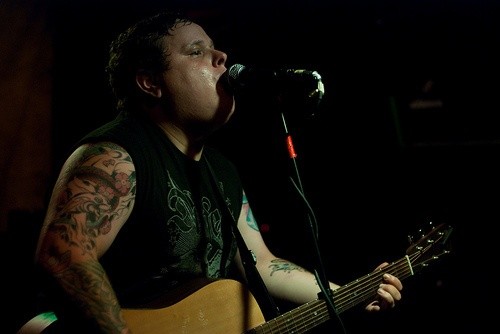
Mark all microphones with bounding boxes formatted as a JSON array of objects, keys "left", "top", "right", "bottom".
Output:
[{"left": 228, "top": 64, "right": 322, "bottom": 85}]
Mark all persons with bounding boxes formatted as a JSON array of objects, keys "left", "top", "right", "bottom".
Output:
[{"left": 34, "top": 15, "right": 403, "bottom": 334}]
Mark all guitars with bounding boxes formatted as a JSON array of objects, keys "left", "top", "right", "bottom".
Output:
[{"left": 17, "top": 219, "right": 469, "bottom": 334}]
